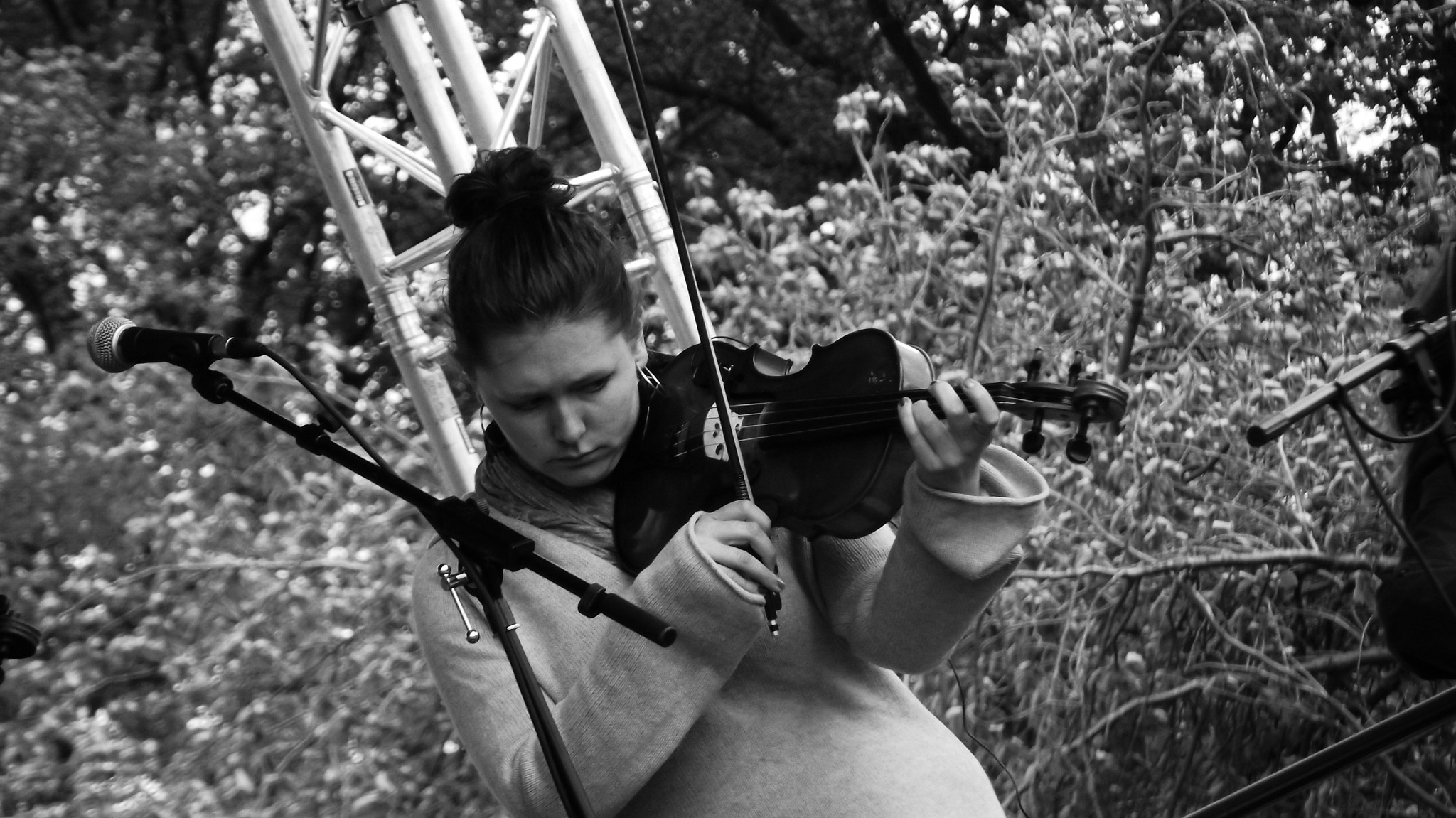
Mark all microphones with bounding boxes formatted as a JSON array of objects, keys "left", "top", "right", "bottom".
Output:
[{"left": 87, "top": 315, "right": 264, "bottom": 373}]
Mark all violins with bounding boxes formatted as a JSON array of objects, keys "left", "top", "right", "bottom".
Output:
[{"left": 610, "top": 326, "right": 1129, "bottom": 577}]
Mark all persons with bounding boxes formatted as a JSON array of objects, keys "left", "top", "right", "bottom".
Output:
[{"left": 408, "top": 146, "right": 1008, "bottom": 818}]
